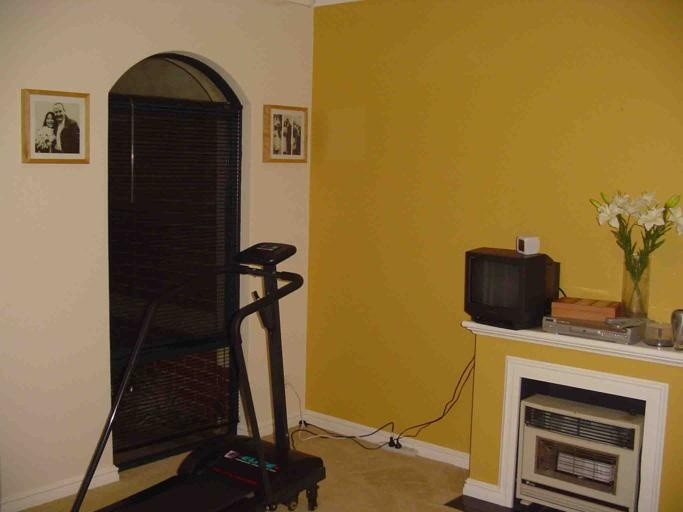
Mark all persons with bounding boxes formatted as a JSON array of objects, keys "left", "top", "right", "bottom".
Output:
[
  {"left": 273, "top": 119, "right": 300, "bottom": 155},
  {"left": 35, "top": 103, "right": 79, "bottom": 153}
]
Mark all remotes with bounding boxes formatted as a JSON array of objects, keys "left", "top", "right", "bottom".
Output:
[{"left": 606, "top": 317, "right": 645, "bottom": 330}]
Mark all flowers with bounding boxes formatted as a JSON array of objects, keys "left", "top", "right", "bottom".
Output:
[{"left": 585, "top": 190, "right": 683, "bottom": 313}]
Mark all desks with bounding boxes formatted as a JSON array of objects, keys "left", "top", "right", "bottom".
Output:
[{"left": 460, "top": 319, "right": 683, "bottom": 511}]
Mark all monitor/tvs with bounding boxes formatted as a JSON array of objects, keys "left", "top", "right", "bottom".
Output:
[{"left": 464, "top": 247, "right": 561, "bottom": 330}]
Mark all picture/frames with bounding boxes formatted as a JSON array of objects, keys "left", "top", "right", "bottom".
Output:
[
  {"left": 20, "top": 88, "right": 91, "bottom": 165},
  {"left": 263, "top": 104, "right": 309, "bottom": 164}
]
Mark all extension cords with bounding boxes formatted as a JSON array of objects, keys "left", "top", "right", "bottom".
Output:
[{"left": 382, "top": 441, "right": 416, "bottom": 457}]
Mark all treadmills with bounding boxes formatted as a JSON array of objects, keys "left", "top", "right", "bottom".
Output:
[{"left": 71, "top": 241, "right": 326, "bottom": 511}]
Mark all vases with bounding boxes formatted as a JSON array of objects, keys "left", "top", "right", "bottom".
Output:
[{"left": 619, "top": 254, "right": 651, "bottom": 318}]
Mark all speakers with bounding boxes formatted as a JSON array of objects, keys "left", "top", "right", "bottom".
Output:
[{"left": 516, "top": 235, "right": 539, "bottom": 256}]
